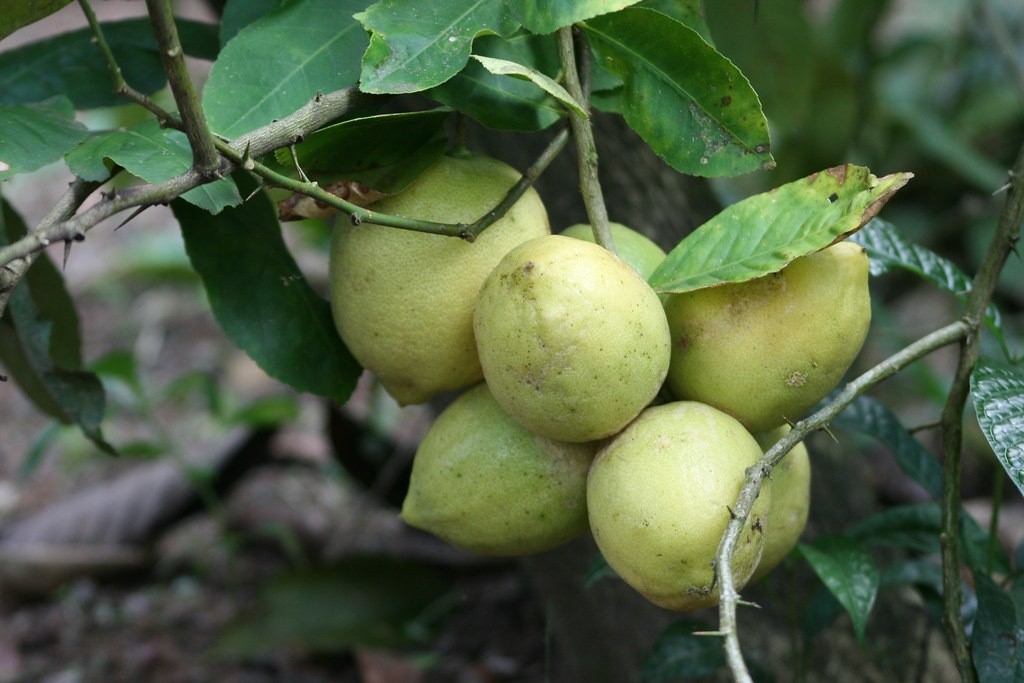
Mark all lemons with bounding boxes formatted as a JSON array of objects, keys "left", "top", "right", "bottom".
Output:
[{"left": 328, "top": 155, "right": 872, "bottom": 615}]
